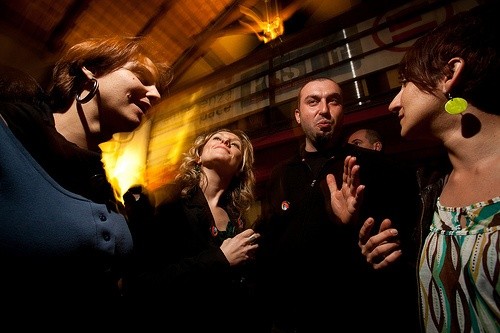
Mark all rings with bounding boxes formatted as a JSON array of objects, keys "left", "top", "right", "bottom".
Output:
[{"left": 246, "top": 236, "right": 257, "bottom": 243}]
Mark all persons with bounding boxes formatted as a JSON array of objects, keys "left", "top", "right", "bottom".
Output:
[
  {"left": 0, "top": 32, "right": 173, "bottom": 333},
  {"left": 123, "top": 20, "right": 500, "bottom": 333}
]
